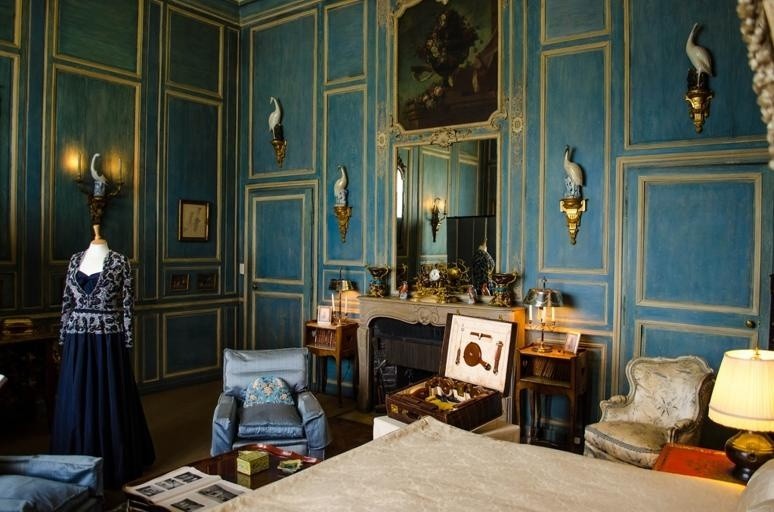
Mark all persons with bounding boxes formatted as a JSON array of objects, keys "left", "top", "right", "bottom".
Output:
[
  {"left": 49, "top": 237, "right": 157, "bottom": 491},
  {"left": 469, "top": 245, "right": 497, "bottom": 293}
]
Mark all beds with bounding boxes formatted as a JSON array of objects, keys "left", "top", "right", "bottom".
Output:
[{"left": 197, "top": 415, "right": 774, "bottom": 512}]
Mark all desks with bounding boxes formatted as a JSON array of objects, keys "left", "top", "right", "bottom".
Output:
[
  {"left": 357, "top": 293, "right": 528, "bottom": 443},
  {"left": 303, "top": 319, "right": 358, "bottom": 409}
]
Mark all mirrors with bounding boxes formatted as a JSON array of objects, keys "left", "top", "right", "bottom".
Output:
[{"left": 390, "top": 133, "right": 501, "bottom": 296}]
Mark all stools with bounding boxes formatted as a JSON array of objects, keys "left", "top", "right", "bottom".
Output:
[{"left": 372, "top": 414, "right": 520, "bottom": 443}]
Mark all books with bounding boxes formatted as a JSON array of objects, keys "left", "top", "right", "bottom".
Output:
[{"left": 124, "top": 464, "right": 252, "bottom": 511}]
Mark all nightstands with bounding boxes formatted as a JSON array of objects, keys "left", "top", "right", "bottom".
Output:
[{"left": 652, "top": 443, "right": 754, "bottom": 486}]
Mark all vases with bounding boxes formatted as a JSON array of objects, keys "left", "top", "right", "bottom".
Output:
[
  {"left": 486, "top": 269, "right": 518, "bottom": 307},
  {"left": 367, "top": 263, "right": 391, "bottom": 297}
]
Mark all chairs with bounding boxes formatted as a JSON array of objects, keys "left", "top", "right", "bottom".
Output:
[
  {"left": 584, "top": 355, "right": 717, "bottom": 469},
  {"left": 209, "top": 348, "right": 333, "bottom": 461},
  {"left": 0, "top": 455, "right": 103, "bottom": 512}
]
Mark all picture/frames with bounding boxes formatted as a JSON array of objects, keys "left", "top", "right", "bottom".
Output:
[
  {"left": 562, "top": 332, "right": 581, "bottom": 354},
  {"left": 317, "top": 305, "right": 333, "bottom": 324},
  {"left": 168, "top": 199, "right": 221, "bottom": 295},
  {"left": 390, "top": 0, "right": 508, "bottom": 134}
]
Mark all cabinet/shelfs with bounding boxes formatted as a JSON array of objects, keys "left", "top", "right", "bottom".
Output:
[
  {"left": 0, "top": 326, "right": 59, "bottom": 431},
  {"left": 516, "top": 342, "right": 592, "bottom": 452}
]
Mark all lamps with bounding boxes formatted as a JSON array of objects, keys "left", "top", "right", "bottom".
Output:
[
  {"left": 328, "top": 268, "right": 354, "bottom": 326},
  {"left": 423, "top": 194, "right": 447, "bottom": 241},
  {"left": 708, "top": 346, "right": 774, "bottom": 481},
  {"left": 239, "top": 255, "right": 245, "bottom": 278},
  {"left": 559, "top": 21, "right": 715, "bottom": 245},
  {"left": 267, "top": 96, "right": 350, "bottom": 241},
  {"left": 75, "top": 151, "right": 124, "bottom": 225},
  {"left": 523, "top": 275, "right": 563, "bottom": 352}
]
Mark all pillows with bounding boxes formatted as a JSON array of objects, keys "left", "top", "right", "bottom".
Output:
[
  {"left": 736, "top": 459, "right": 774, "bottom": 512},
  {"left": 243, "top": 376, "right": 296, "bottom": 406}
]
